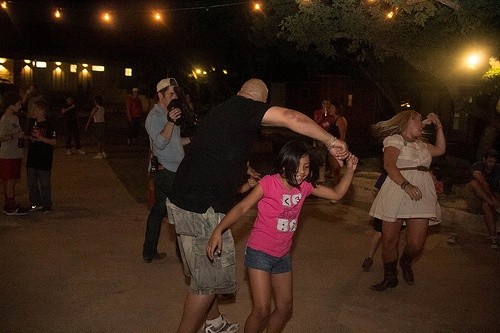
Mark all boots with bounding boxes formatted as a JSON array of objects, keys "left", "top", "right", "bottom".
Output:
[
  {"left": 361, "top": 257, "right": 374, "bottom": 272},
  {"left": 370, "top": 261, "right": 399, "bottom": 291},
  {"left": 399, "top": 252, "right": 415, "bottom": 286}
]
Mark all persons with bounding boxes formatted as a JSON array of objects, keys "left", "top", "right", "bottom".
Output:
[
  {"left": 362, "top": 110, "right": 445, "bottom": 292},
  {"left": 61, "top": 94, "right": 86, "bottom": 154},
  {"left": 125, "top": 87, "right": 143, "bottom": 147},
  {"left": 143, "top": 78, "right": 195, "bottom": 262},
  {"left": 85, "top": 95, "right": 108, "bottom": 159},
  {"left": 206, "top": 139, "right": 359, "bottom": 333},
  {"left": 311, "top": 97, "right": 347, "bottom": 204},
  {"left": 164, "top": 78, "right": 349, "bottom": 333},
  {"left": 236, "top": 150, "right": 275, "bottom": 192},
  {"left": 462, "top": 149, "right": 500, "bottom": 249},
  {"left": 0, "top": 84, "right": 57, "bottom": 216}
]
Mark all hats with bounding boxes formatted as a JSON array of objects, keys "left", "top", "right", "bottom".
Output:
[
  {"left": 156, "top": 77, "right": 179, "bottom": 93},
  {"left": 132, "top": 88, "right": 138, "bottom": 91}
]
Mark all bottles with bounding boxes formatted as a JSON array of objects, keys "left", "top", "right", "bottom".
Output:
[
  {"left": 325, "top": 107, "right": 327, "bottom": 116},
  {"left": 34, "top": 121, "right": 39, "bottom": 139}
]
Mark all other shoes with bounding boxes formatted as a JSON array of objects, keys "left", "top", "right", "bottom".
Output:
[
  {"left": 76, "top": 149, "right": 86, "bottom": 155},
  {"left": 103, "top": 152, "right": 109, "bottom": 158},
  {"left": 202, "top": 318, "right": 239, "bottom": 333},
  {"left": 43, "top": 207, "right": 52, "bottom": 214},
  {"left": 27, "top": 203, "right": 42, "bottom": 212},
  {"left": 66, "top": 148, "right": 72, "bottom": 155},
  {"left": 145, "top": 251, "right": 167, "bottom": 263},
  {"left": 447, "top": 232, "right": 459, "bottom": 244},
  {"left": 2, "top": 207, "right": 28, "bottom": 216},
  {"left": 488, "top": 236, "right": 498, "bottom": 248},
  {"left": 93, "top": 155, "right": 103, "bottom": 159}
]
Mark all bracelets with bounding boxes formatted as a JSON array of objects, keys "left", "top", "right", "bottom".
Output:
[
  {"left": 402, "top": 181, "right": 409, "bottom": 189},
  {"left": 326, "top": 137, "right": 337, "bottom": 151}
]
